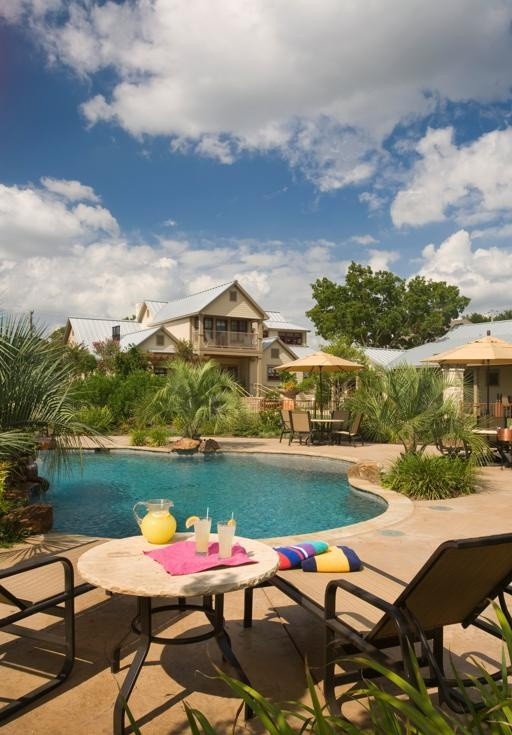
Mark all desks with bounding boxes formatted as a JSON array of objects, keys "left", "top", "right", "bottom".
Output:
[{"left": 78, "top": 531, "right": 280, "bottom": 735}]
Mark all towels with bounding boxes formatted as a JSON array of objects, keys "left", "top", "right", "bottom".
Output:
[
  {"left": 301, "top": 546, "right": 361, "bottom": 573},
  {"left": 273, "top": 539, "right": 329, "bottom": 571}
]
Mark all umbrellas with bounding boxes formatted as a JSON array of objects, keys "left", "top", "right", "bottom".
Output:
[
  {"left": 272, "top": 350, "right": 366, "bottom": 441},
  {"left": 419, "top": 328, "right": 512, "bottom": 456}
]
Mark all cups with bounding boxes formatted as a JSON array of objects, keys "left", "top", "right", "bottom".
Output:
[
  {"left": 193, "top": 517, "right": 212, "bottom": 557},
  {"left": 216, "top": 520, "right": 236, "bottom": 560}
]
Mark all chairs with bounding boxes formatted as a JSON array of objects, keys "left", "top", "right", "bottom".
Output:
[
  {"left": 244, "top": 532, "right": 512, "bottom": 735},
  {"left": 278, "top": 410, "right": 364, "bottom": 449},
  {"left": 432, "top": 416, "right": 512, "bottom": 468},
  {"left": 1, "top": 538, "right": 114, "bottom": 722}
]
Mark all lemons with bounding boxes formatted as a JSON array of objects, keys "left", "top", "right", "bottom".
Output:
[
  {"left": 227, "top": 519, "right": 236, "bottom": 526},
  {"left": 185, "top": 516, "right": 199, "bottom": 528}
]
